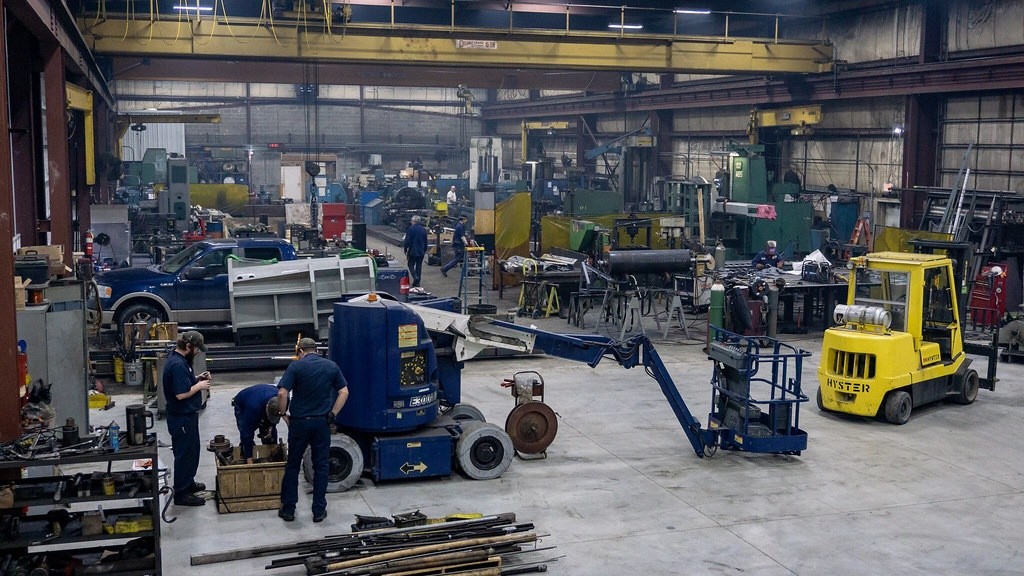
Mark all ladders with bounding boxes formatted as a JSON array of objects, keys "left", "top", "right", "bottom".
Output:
[
  {"left": 458, "top": 246, "right": 489, "bottom": 316},
  {"left": 844, "top": 216, "right": 872, "bottom": 259}
]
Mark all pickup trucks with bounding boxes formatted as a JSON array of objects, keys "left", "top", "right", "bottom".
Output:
[{"left": 85, "top": 238, "right": 411, "bottom": 344}]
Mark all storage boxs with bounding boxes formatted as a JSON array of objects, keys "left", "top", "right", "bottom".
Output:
[
  {"left": 14, "top": 253, "right": 51, "bottom": 280},
  {"left": 15, "top": 260, "right": 49, "bottom": 283},
  {"left": 14, "top": 276, "right": 31, "bottom": 311},
  {"left": 217, "top": 442, "right": 287, "bottom": 512},
  {"left": 124, "top": 321, "right": 146, "bottom": 349},
  {"left": 21, "top": 245, "right": 64, "bottom": 263},
  {"left": 157, "top": 321, "right": 178, "bottom": 345}
]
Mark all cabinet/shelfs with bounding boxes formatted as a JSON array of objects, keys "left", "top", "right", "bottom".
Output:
[{"left": 0, "top": 432, "right": 161, "bottom": 575}]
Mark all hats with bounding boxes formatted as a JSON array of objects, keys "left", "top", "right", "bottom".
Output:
[
  {"left": 266, "top": 397, "right": 281, "bottom": 423},
  {"left": 296, "top": 338, "right": 316, "bottom": 356},
  {"left": 451, "top": 186, "right": 455, "bottom": 188},
  {"left": 767, "top": 240, "right": 777, "bottom": 248},
  {"left": 183, "top": 331, "right": 208, "bottom": 352}
]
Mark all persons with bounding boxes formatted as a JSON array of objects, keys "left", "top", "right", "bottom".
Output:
[
  {"left": 441, "top": 215, "right": 473, "bottom": 277},
  {"left": 278, "top": 338, "right": 348, "bottom": 522},
  {"left": 404, "top": 216, "right": 428, "bottom": 286},
  {"left": 234, "top": 384, "right": 291, "bottom": 464},
  {"left": 447, "top": 186, "right": 459, "bottom": 205},
  {"left": 751, "top": 241, "right": 784, "bottom": 269},
  {"left": 163, "top": 331, "right": 212, "bottom": 506}
]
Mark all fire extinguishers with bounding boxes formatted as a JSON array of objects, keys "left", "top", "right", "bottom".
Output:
[
  {"left": 400, "top": 271, "right": 409, "bottom": 294},
  {"left": 85, "top": 229, "right": 95, "bottom": 255}
]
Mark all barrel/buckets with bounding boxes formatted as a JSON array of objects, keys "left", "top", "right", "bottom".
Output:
[{"left": 113, "top": 354, "right": 144, "bottom": 386}]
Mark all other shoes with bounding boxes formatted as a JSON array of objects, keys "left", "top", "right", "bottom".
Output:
[
  {"left": 440, "top": 268, "right": 448, "bottom": 277},
  {"left": 313, "top": 510, "right": 327, "bottom": 522},
  {"left": 193, "top": 482, "right": 206, "bottom": 494},
  {"left": 175, "top": 494, "right": 206, "bottom": 506},
  {"left": 412, "top": 278, "right": 419, "bottom": 287},
  {"left": 278, "top": 513, "right": 294, "bottom": 521}
]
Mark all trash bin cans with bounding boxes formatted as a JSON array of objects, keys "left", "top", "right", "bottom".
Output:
[{"left": 352, "top": 223, "right": 366, "bottom": 252}]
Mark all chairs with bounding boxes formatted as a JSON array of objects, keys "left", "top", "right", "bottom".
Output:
[{"left": 887, "top": 307, "right": 904, "bottom": 333}]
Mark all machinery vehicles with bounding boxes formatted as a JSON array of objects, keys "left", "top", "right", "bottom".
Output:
[
  {"left": 816, "top": 249, "right": 1003, "bottom": 424},
  {"left": 301, "top": 292, "right": 814, "bottom": 494}
]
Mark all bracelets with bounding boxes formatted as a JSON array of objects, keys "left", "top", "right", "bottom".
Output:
[{"left": 279, "top": 412, "right": 286, "bottom": 416}]
